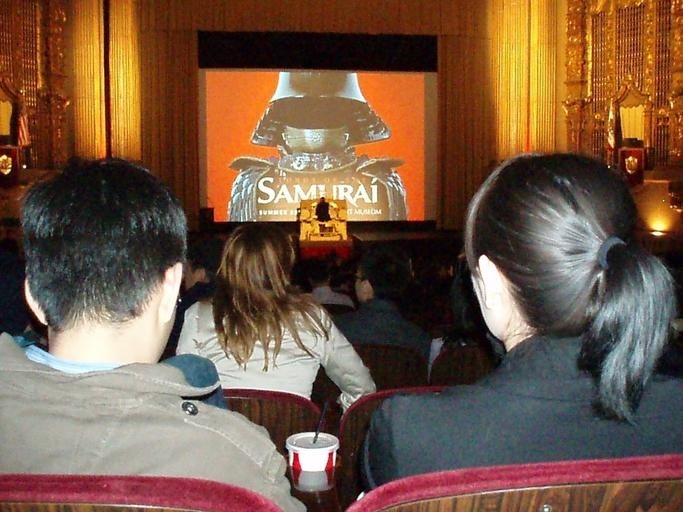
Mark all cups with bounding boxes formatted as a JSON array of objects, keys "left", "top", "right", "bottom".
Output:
[
  {"left": 282, "top": 430, "right": 341, "bottom": 470},
  {"left": 290, "top": 470, "right": 339, "bottom": 495}
]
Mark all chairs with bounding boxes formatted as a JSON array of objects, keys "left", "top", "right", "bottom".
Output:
[
  {"left": 221, "top": 388, "right": 325, "bottom": 457},
  {"left": 344, "top": 454, "right": 682, "bottom": 512},
  {"left": 339, "top": 345, "right": 494, "bottom": 457},
  {"left": 0, "top": 473, "right": 282, "bottom": 512}
]
